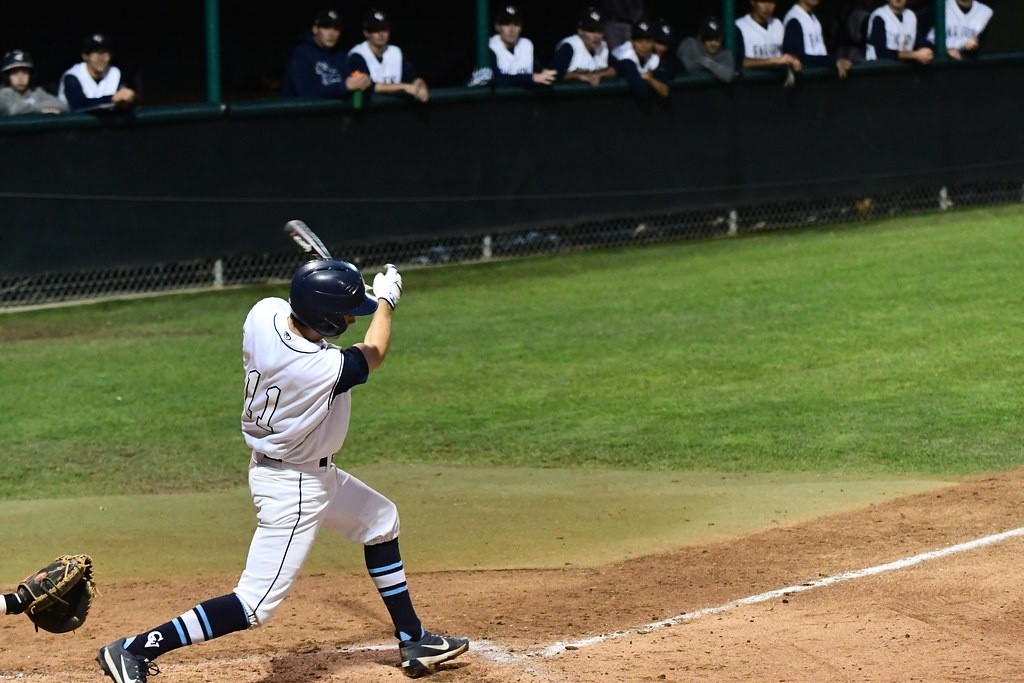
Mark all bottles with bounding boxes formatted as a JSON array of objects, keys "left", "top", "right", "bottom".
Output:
[{"left": 353, "top": 69, "right": 363, "bottom": 108}]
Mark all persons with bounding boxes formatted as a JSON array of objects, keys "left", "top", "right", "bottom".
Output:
[
  {"left": 0, "top": 49, "right": 68, "bottom": 116},
  {"left": 924, "top": 0, "right": 994, "bottom": 60},
  {"left": 466, "top": 5, "right": 557, "bottom": 86},
  {"left": 865, "top": 0, "right": 933, "bottom": 64},
  {"left": 58, "top": 33, "right": 135, "bottom": 112},
  {"left": 605, "top": 12, "right": 736, "bottom": 103},
  {"left": 734, "top": 0, "right": 802, "bottom": 86},
  {"left": 97, "top": 257, "right": 469, "bottom": 683},
  {"left": 782, "top": 0, "right": 852, "bottom": 77},
  {"left": 553, "top": 9, "right": 621, "bottom": 88},
  {"left": 0, "top": 554, "right": 96, "bottom": 633},
  {"left": 347, "top": 12, "right": 429, "bottom": 103},
  {"left": 284, "top": 10, "right": 375, "bottom": 100}
]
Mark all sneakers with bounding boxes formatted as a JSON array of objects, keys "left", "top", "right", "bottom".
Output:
[
  {"left": 94, "top": 638, "right": 159, "bottom": 683},
  {"left": 398, "top": 631, "right": 469, "bottom": 679}
]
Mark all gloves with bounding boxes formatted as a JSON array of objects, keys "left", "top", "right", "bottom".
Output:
[{"left": 373, "top": 263, "right": 403, "bottom": 310}]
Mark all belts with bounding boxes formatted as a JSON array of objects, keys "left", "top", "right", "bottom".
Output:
[{"left": 263, "top": 453, "right": 334, "bottom": 467}]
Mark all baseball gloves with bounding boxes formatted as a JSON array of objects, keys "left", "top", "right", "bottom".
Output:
[{"left": 17, "top": 554, "right": 94, "bottom": 634}]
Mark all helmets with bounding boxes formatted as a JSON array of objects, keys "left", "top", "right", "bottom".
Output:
[{"left": 288, "top": 260, "right": 379, "bottom": 338}]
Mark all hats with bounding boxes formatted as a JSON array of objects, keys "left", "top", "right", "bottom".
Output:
[
  {"left": 495, "top": 6, "right": 522, "bottom": 23},
  {"left": 82, "top": 32, "right": 112, "bottom": 53},
  {"left": 699, "top": 17, "right": 723, "bottom": 37},
  {"left": 653, "top": 19, "right": 674, "bottom": 42},
  {"left": 630, "top": 21, "right": 653, "bottom": 40},
  {"left": 0, "top": 49, "right": 33, "bottom": 72},
  {"left": 313, "top": 10, "right": 345, "bottom": 31},
  {"left": 574, "top": 6, "right": 606, "bottom": 30},
  {"left": 361, "top": 10, "right": 391, "bottom": 31}
]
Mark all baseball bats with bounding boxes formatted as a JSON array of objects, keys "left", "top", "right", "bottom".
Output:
[{"left": 284, "top": 220, "right": 332, "bottom": 261}]
